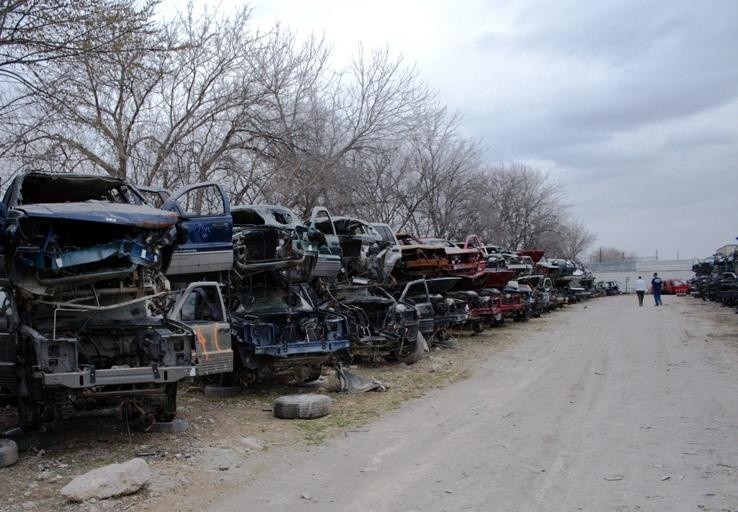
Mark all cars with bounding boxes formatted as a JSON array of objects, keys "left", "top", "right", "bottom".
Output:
[
  {"left": 0, "top": 168, "right": 620, "bottom": 430},
  {"left": 647, "top": 249, "right": 738, "bottom": 314}
]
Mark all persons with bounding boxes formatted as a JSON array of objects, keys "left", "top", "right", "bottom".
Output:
[
  {"left": 650, "top": 272, "right": 663, "bottom": 307},
  {"left": 634, "top": 275, "right": 648, "bottom": 307}
]
